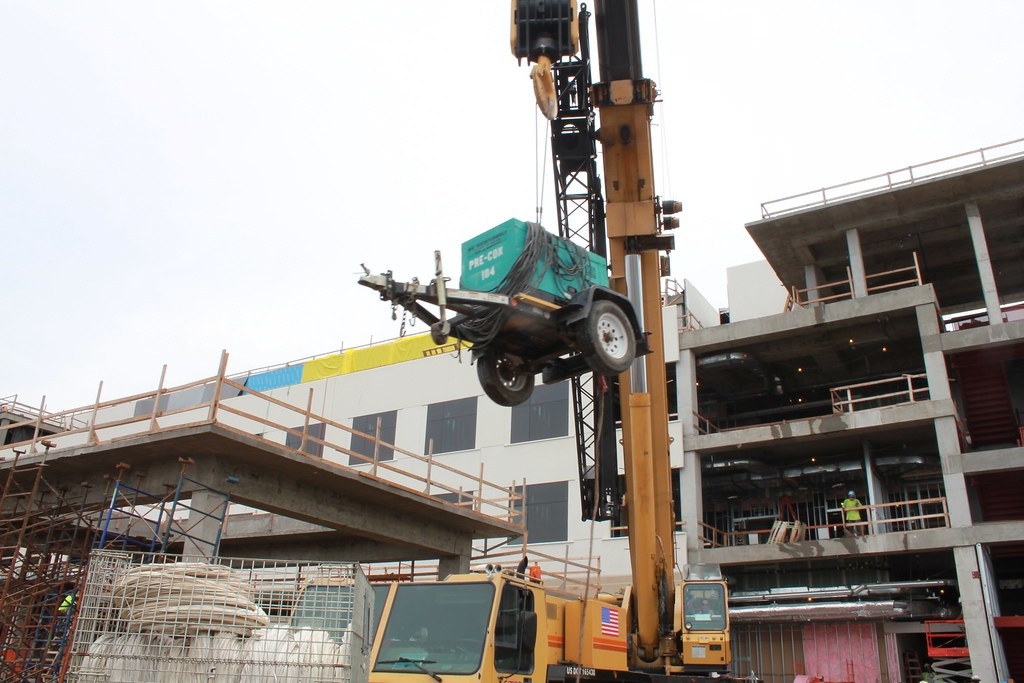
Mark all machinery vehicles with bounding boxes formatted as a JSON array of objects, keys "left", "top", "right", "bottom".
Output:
[{"left": 349, "top": 0, "right": 734, "bottom": 683}]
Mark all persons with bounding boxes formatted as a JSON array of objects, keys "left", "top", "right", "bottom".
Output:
[
  {"left": 842, "top": 490, "right": 865, "bottom": 537},
  {"left": 53, "top": 592, "right": 74, "bottom": 640}
]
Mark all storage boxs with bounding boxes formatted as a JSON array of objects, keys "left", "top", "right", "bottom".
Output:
[{"left": 460, "top": 218, "right": 610, "bottom": 306}]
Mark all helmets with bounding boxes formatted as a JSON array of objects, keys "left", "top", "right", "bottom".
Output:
[{"left": 848, "top": 490, "right": 856, "bottom": 497}]
